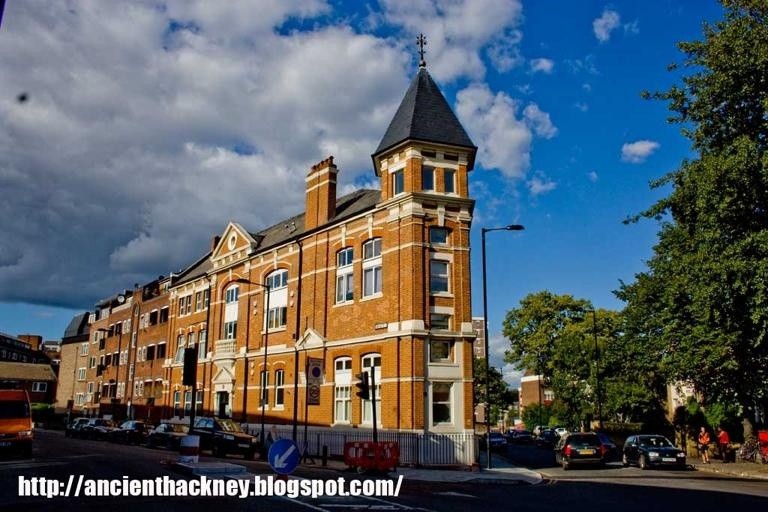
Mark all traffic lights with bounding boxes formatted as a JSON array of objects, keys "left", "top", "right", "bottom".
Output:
[{"left": 354, "top": 371, "right": 369, "bottom": 401}]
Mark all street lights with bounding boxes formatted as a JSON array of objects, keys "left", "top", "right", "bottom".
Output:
[
  {"left": 569, "top": 307, "right": 604, "bottom": 429},
  {"left": 235, "top": 278, "right": 270, "bottom": 461},
  {"left": 481, "top": 225, "right": 525, "bottom": 469},
  {"left": 97, "top": 328, "right": 122, "bottom": 423}
]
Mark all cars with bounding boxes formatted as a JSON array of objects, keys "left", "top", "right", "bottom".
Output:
[
  {"left": 64, "top": 417, "right": 255, "bottom": 461},
  {"left": 480, "top": 425, "right": 685, "bottom": 470}
]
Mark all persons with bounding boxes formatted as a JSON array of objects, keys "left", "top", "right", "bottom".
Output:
[
  {"left": 717, "top": 425, "right": 731, "bottom": 461},
  {"left": 697, "top": 426, "right": 710, "bottom": 464}
]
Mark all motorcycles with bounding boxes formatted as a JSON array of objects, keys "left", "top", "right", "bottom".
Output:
[{"left": 739, "top": 436, "right": 768, "bottom": 464}]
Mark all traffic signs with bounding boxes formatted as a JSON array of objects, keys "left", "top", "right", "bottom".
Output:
[{"left": 307, "top": 383, "right": 320, "bottom": 405}]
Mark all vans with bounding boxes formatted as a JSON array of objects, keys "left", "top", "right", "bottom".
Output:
[{"left": 0, "top": 390, "right": 34, "bottom": 453}]
